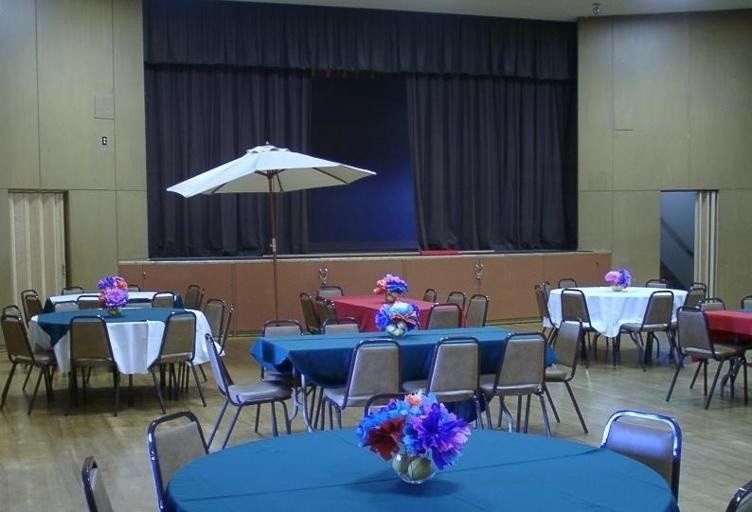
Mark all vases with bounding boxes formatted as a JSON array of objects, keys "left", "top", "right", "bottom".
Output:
[{"left": 392, "top": 453, "right": 436, "bottom": 485}]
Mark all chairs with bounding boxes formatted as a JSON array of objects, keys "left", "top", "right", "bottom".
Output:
[
  {"left": 725, "top": 480, "right": 752, "bottom": 512},
  {"left": 82, "top": 456, "right": 116, "bottom": 512},
  {"left": 600, "top": 410, "right": 682, "bottom": 503},
  {"left": 147, "top": 410, "right": 210, "bottom": 511}
]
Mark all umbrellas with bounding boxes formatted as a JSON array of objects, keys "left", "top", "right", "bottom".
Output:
[{"left": 167, "top": 141, "right": 376, "bottom": 322}]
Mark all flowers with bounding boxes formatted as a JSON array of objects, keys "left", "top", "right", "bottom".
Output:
[
  {"left": 604, "top": 268, "right": 631, "bottom": 288},
  {"left": 355, "top": 388, "right": 473, "bottom": 481}
]
